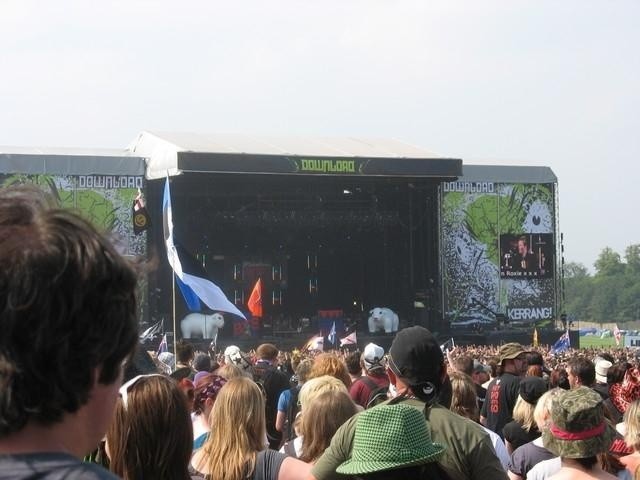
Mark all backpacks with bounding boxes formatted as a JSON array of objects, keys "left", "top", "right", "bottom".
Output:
[
  {"left": 246, "top": 365, "right": 276, "bottom": 415},
  {"left": 359, "top": 376, "right": 389, "bottom": 410}
]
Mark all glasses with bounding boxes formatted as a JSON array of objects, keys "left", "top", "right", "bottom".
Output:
[{"left": 118, "top": 374, "right": 161, "bottom": 411}]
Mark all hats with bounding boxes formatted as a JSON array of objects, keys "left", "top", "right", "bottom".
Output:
[
  {"left": 591, "top": 356, "right": 613, "bottom": 384},
  {"left": 473, "top": 359, "right": 492, "bottom": 372},
  {"left": 336, "top": 404, "right": 447, "bottom": 475},
  {"left": 194, "top": 355, "right": 210, "bottom": 371},
  {"left": 158, "top": 352, "right": 176, "bottom": 367},
  {"left": 383, "top": 326, "right": 445, "bottom": 402},
  {"left": 363, "top": 342, "right": 386, "bottom": 371},
  {"left": 609, "top": 368, "right": 640, "bottom": 413},
  {"left": 495, "top": 342, "right": 532, "bottom": 366},
  {"left": 519, "top": 376, "right": 548, "bottom": 404},
  {"left": 542, "top": 386, "right": 618, "bottom": 459},
  {"left": 192, "top": 371, "right": 209, "bottom": 385}
]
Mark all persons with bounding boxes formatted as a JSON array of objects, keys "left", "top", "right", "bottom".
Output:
[
  {"left": 508, "top": 235, "right": 540, "bottom": 273},
  {"left": 82, "top": 326, "right": 640, "bottom": 480},
  {"left": 0, "top": 186, "right": 139, "bottom": 479}
]
